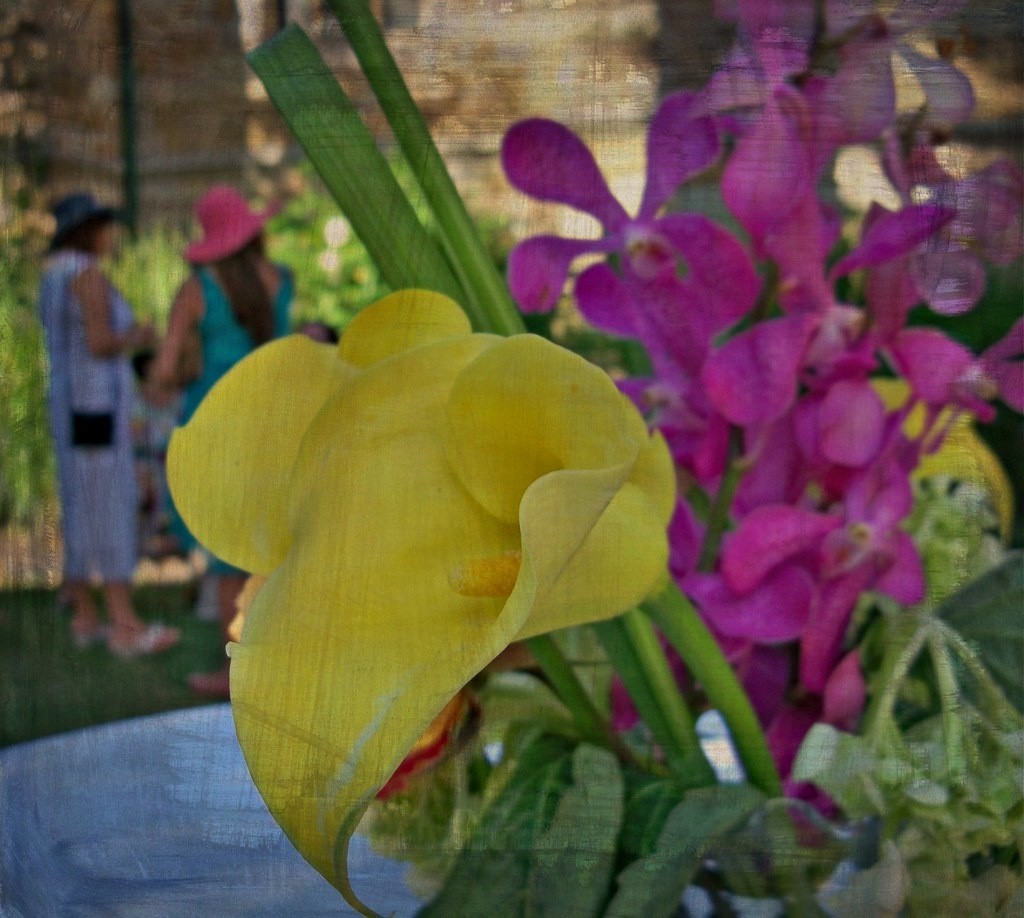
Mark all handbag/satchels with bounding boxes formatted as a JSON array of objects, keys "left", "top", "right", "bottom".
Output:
[{"left": 71, "top": 410, "right": 116, "bottom": 450}]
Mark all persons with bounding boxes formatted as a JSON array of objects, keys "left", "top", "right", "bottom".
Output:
[
  {"left": 129, "top": 331, "right": 183, "bottom": 459},
  {"left": 295, "top": 319, "right": 340, "bottom": 345},
  {"left": 32, "top": 189, "right": 180, "bottom": 656},
  {"left": 154, "top": 185, "right": 302, "bottom": 632}
]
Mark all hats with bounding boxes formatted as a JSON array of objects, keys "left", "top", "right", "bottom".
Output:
[
  {"left": 48, "top": 191, "right": 119, "bottom": 251},
  {"left": 185, "top": 187, "right": 281, "bottom": 262}
]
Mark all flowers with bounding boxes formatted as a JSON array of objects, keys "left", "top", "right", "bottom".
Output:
[{"left": 168, "top": 0, "right": 1024, "bottom": 918}]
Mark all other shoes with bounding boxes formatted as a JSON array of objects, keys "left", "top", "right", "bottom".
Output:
[
  {"left": 110, "top": 626, "right": 178, "bottom": 661},
  {"left": 189, "top": 661, "right": 229, "bottom": 701},
  {"left": 74, "top": 623, "right": 107, "bottom": 651}
]
